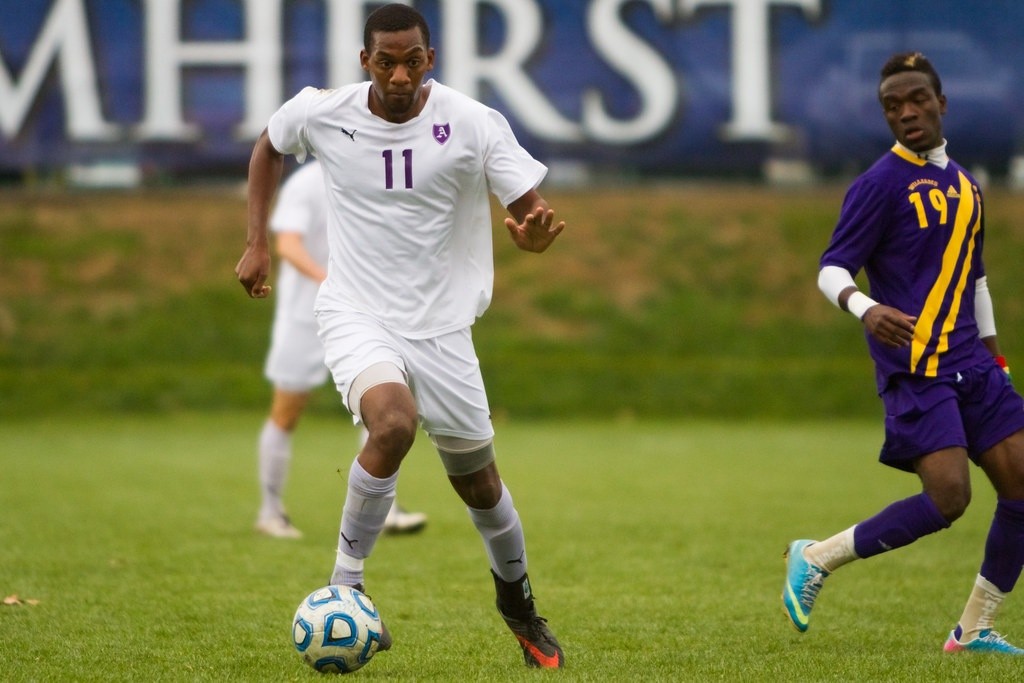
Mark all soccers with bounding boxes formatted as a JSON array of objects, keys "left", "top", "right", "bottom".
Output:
[{"left": 292, "top": 585, "right": 383, "bottom": 674}]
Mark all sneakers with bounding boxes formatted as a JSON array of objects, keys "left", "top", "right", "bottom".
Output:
[
  {"left": 329, "top": 578, "right": 393, "bottom": 654},
  {"left": 380, "top": 507, "right": 427, "bottom": 534},
  {"left": 495, "top": 602, "right": 568, "bottom": 675},
  {"left": 257, "top": 517, "right": 306, "bottom": 541},
  {"left": 781, "top": 536, "right": 831, "bottom": 633},
  {"left": 942, "top": 626, "right": 1023, "bottom": 660}
]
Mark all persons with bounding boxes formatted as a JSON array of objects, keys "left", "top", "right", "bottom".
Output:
[
  {"left": 235, "top": 3, "right": 567, "bottom": 674},
  {"left": 776, "top": 54, "right": 1024, "bottom": 656},
  {"left": 253, "top": 157, "right": 429, "bottom": 539}
]
